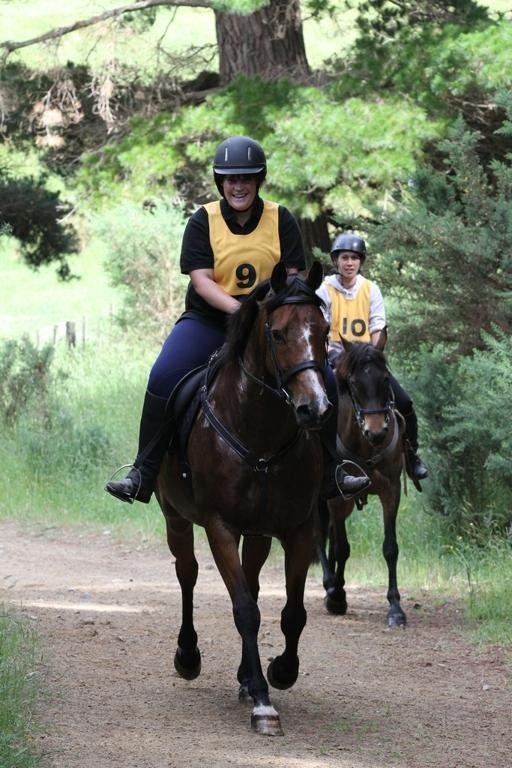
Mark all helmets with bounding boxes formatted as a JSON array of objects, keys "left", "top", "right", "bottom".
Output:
[
  {"left": 212, "top": 135, "right": 266, "bottom": 198},
  {"left": 329, "top": 232, "right": 368, "bottom": 261}
]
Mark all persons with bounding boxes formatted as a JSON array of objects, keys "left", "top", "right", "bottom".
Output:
[
  {"left": 314, "top": 232, "right": 429, "bottom": 480},
  {"left": 105, "top": 134, "right": 370, "bottom": 505}
]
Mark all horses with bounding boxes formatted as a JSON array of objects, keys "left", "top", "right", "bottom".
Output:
[
  {"left": 307, "top": 324, "right": 408, "bottom": 632},
  {"left": 150, "top": 257, "right": 333, "bottom": 738}
]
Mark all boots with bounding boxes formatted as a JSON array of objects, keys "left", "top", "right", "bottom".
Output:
[
  {"left": 312, "top": 399, "right": 374, "bottom": 496},
  {"left": 102, "top": 387, "right": 178, "bottom": 505},
  {"left": 397, "top": 409, "right": 430, "bottom": 482}
]
